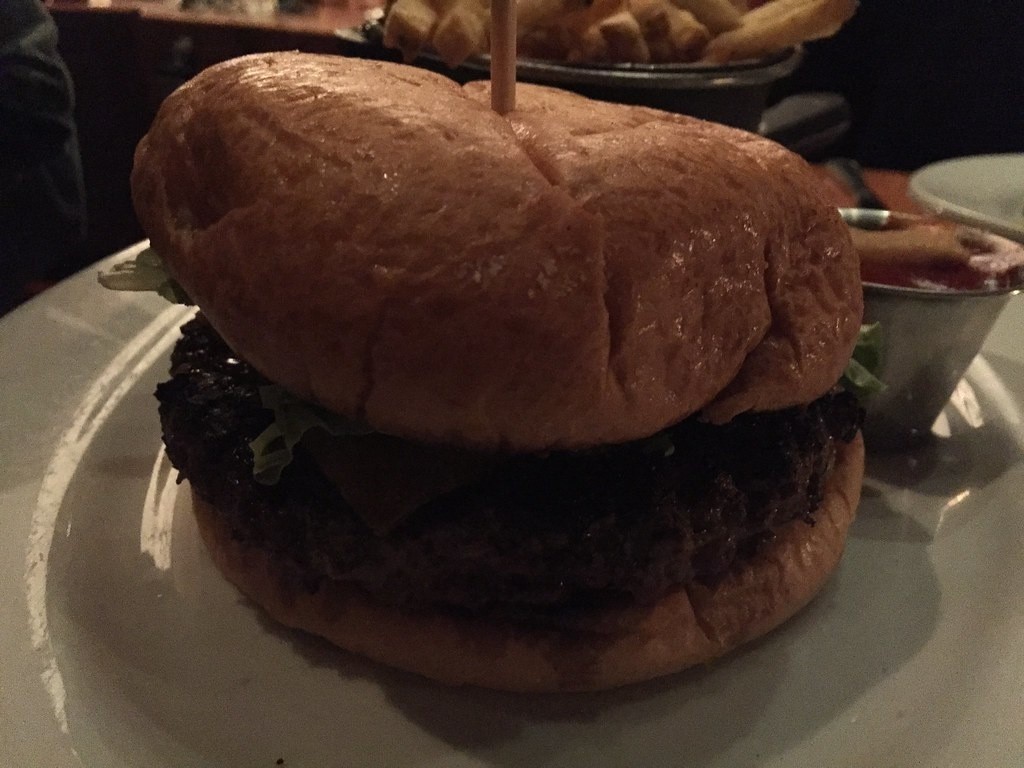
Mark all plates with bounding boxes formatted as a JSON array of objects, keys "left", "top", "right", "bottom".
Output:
[
  {"left": 0, "top": 237, "right": 1024, "bottom": 768},
  {"left": 907, "top": 152, "right": 1024, "bottom": 241}
]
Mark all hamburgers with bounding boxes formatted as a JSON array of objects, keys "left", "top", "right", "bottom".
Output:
[{"left": 96, "top": 53, "right": 887, "bottom": 694}]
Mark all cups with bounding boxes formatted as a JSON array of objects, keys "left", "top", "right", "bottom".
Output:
[{"left": 836, "top": 209, "right": 1024, "bottom": 431}]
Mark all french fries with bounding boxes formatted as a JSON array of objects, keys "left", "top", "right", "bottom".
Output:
[{"left": 380, "top": 0, "right": 855, "bottom": 64}]
